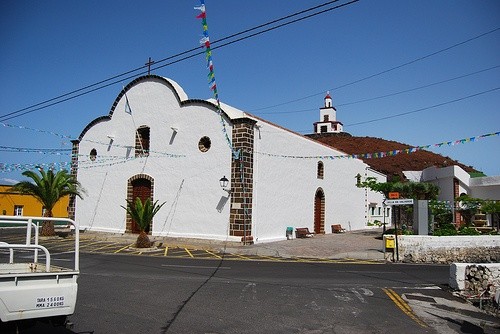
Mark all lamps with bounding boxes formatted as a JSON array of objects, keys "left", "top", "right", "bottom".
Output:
[{"left": 219, "top": 176, "right": 230, "bottom": 188}]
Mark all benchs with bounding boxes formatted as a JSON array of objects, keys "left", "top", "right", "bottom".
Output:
[
  {"left": 295, "top": 228, "right": 316, "bottom": 239},
  {"left": 331, "top": 224, "right": 346, "bottom": 234}
]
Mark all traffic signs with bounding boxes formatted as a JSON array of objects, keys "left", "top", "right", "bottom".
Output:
[{"left": 383, "top": 199, "right": 415, "bottom": 205}]
